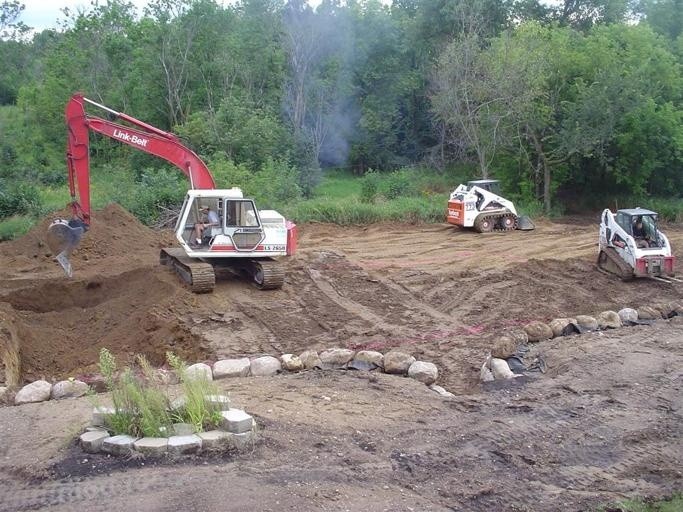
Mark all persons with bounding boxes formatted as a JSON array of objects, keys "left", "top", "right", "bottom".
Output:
[
  {"left": 606, "top": 219, "right": 627, "bottom": 249},
  {"left": 191, "top": 205, "right": 221, "bottom": 250},
  {"left": 633, "top": 218, "right": 655, "bottom": 249}
]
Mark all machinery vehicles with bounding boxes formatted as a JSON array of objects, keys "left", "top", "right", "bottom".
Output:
[
  {"left": 444, "top": 177, "right": 533, "bottom": 235},
  {"left": 592, "top": 205, "right": 682, "bottom": 285},
  {"left": 45, "top": 89, "right": 301, "bottom": 297}
]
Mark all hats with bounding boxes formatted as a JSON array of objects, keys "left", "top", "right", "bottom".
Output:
[{"left": 198, "top": 205, "right": 208, "bottom": 211}]
